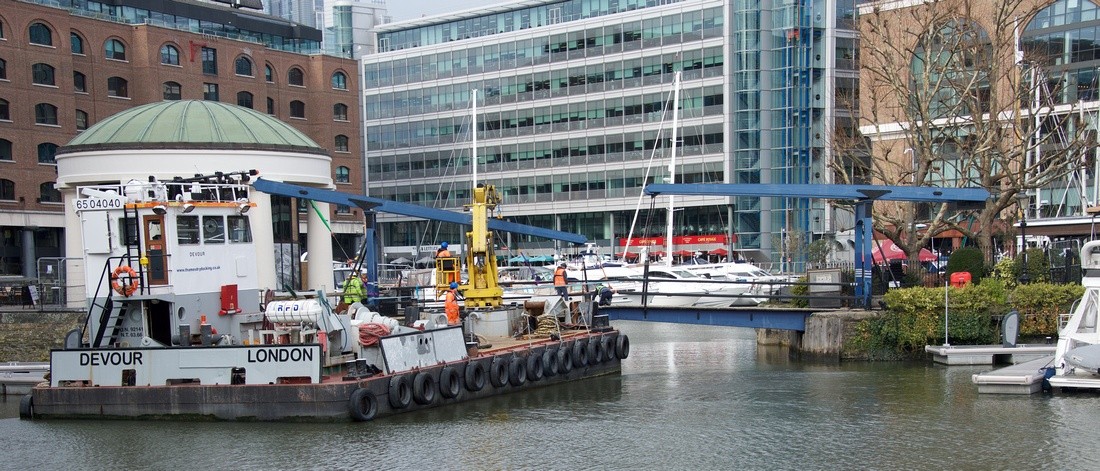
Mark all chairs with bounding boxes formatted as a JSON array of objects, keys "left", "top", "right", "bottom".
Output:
[{"left": 0, "top": 286, "right": 22, "bottom": 306}]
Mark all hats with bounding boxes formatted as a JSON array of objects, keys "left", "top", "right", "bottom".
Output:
[{"left": 561, "top": 263, "right": 567, "bottom": 268}]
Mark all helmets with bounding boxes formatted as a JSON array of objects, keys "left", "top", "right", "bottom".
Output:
[
  {"left": 450, "top": 283, "right": 458, "bottom": 288},
  {"left": 442, "top": 242, "right": 447, "bottom": 248},
  {"left": 349, "top": 271, "right": 356, "bottom": 275},
  {"left": 362, "top": 269, "right": 367, "bottom": 273}
]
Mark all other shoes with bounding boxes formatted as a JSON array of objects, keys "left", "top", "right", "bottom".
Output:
[
  {"left": 605, "top": 298, "right": 609, "bottom": 305},
  {"left": 609, "top": 298, "right": 612, "bottom": 304}
]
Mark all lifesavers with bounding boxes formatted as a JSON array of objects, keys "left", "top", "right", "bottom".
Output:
[
  {"left": 348, "top": 388, "right": 378, "bottom": 421},
  {"left": 616, "top": 334, "right": 629, "bottom": 359},
  {"left": 413, "top": 372, "right": 436, "bottom": 405},
  {"left": 112, "top": 267, "right": 137, "bottom": 296},
  {"left": 556, "top": 348, "right": 573, "bottom": 374},
  {"left": 19, "top": 393, "right": 33, "bottom": 420},
  {"left": 586, "top": 341, "right": 604, "bottom": 365},
  {"left": 389, "top": 376, "right": 412, "bottom": 410},
  {"left": 526, "top": 355, "right": 545, "bottom": 381},
  {"left": 573, "top": 341, "right": 589, "bottom": 369},
  {"left": 600, "top": 336, "right": 615, "bottom": 361},
  {"left": 490, "top": 357, "right": 510, "bottom": 388},
  {"left": 543, "top": 349, "right": 560, "bottom": 378},
  {"left": 465, "top": 360, "right": 485, "bottom": 391},
  {"left": 509, "top": 359, "right": 527, "bottom": 386},
  {"left": 439, "top": 367, "right": 461, "bottom": 399}
]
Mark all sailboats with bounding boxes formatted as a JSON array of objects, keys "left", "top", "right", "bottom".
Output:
[
  {"left": 402, "top": 68, "right": 792, "bottom": 307},
  {"left": 1012, "top": 60, "right": 1100, "bottom": 236}
]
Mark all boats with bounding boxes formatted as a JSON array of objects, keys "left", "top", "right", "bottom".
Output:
[
  {"left": 1038, "top": 239, "right": 1100, "bottom": 395},
  {"left": 18, "top": 181, "right": 629, "bottom": 425}
]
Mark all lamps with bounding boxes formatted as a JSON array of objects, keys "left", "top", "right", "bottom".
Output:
[{"left": 153, "top": 202, "right": 252, "bottom": 215}]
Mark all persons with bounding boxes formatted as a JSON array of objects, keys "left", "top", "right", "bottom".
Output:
[
  {"left": 445, "top": 283, "right": 459, "bottom": 325},
  {"left": 591, "top": 286, "right": 612, "bottom": 306},
  {"left": 343, "top": 273, "right": 369, "bottom": 307},
  {"left": 553, "top": 263, "right": 570, "bottom": 302},
  {"left": 436, "top": 242, "right": 450, "bottom": 258}
]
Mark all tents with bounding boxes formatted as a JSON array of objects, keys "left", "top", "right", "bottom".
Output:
[
  {"left": 862, "top": 237, "right": 937, "bottom": 267},
  {"left": 509, "top": 255, "right": 554, "bottom": 268},
  {"left": 390, "top": 256, "right": 435, "bottom": 268}
]
